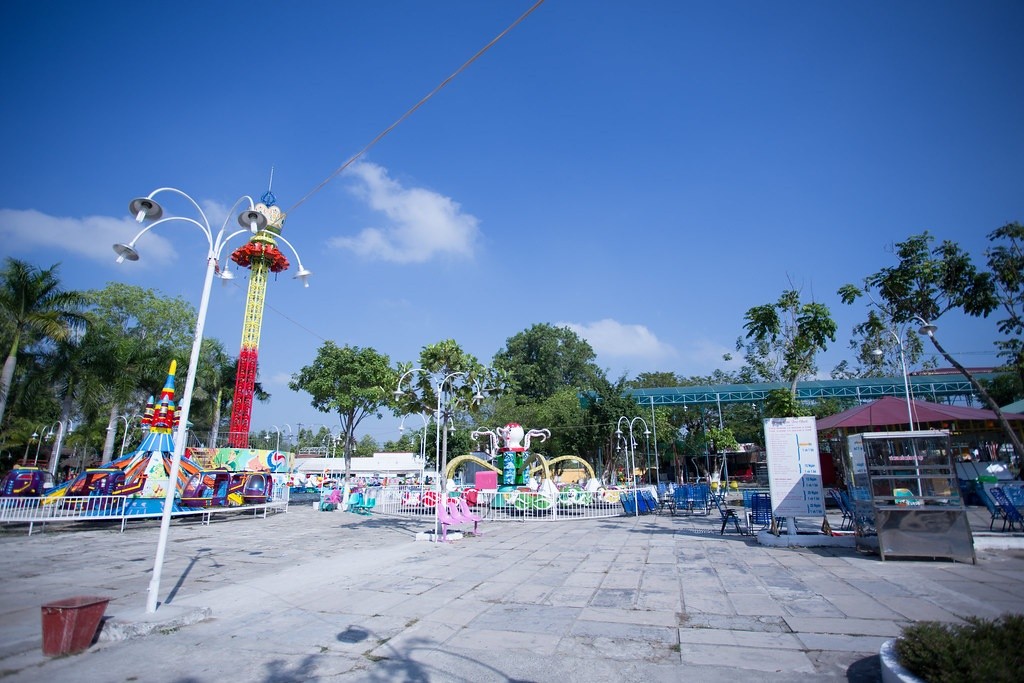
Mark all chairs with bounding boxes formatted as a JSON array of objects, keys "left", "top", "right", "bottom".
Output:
[
  {"left": 975, "top": 479, "right": 1024, "bottom": 534},
  {"left": 320, "top": 489, "right": 376, "bottom": 517},
  {"left": 828, "top": 483, "right": 877, "bottom": 533},
  {"left": 619, "top": 480, "right": 786, "bottom": 540}
]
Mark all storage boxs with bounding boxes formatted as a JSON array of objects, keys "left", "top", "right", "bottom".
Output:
[{"left": 41, "top": 594, "right": 113, "bottom": 657}]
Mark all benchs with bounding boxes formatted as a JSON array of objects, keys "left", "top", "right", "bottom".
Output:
[{"left": 436, "top": 498, "right": 484, "bottom": 544}]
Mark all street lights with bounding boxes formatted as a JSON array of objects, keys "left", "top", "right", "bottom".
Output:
[
  {"left": 617, "top": 434, "right": 638, "bottom": 490},
  {"left": 399, "top": 410, "right": 458, "bottom": 509},
  {"left": 617, "top": 416, "right": 654, "bottom": 517},
  {"left": 106, "top": 413, "right": 143, "bottom": 470},
  {"left": 394, "top": 368, "right": 492, "bottom": 547},
  {"left": 316, "top": 425, "right": 347, "bottom": 460},
  {"left": 31, "top": 423, "right": 52, "bottom": 467},
  {"left": 49, "top": 419, "right": 74, "bottom": 475},
  {"left": 873, "top": 316, "right": 937, "bottom": 505},
  {"left": 115, "top": 186, "right": 311, "bottom": 612},
  {"left": 264, "top": 422, "right": 295, "bottom": 498}
]
